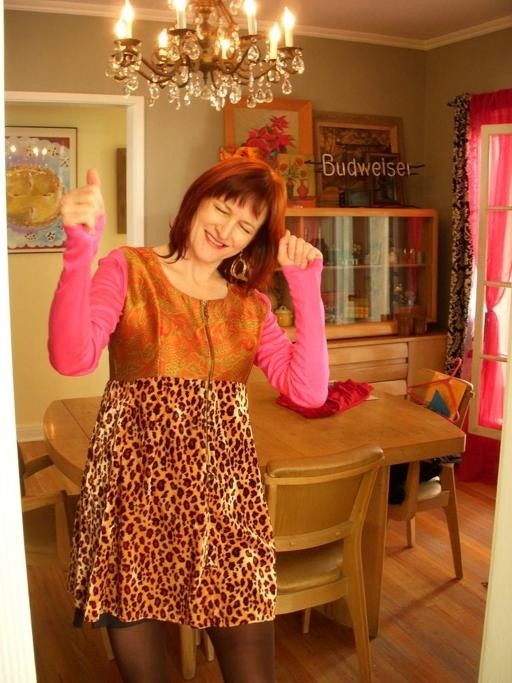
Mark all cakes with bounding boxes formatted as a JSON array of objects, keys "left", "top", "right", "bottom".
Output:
[{"left": 6, "top": 166, "right": 65, "bottom": 230}]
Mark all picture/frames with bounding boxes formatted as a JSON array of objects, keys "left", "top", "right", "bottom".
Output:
[
  {"left": 313, "top": 110, "right": 408, "bottom": 207},
  {"left": 5, "top": 125, "right": 78, "bottom": 254},
  {"left": 224, "top": 94, "right": 314, "bottom": 199}
]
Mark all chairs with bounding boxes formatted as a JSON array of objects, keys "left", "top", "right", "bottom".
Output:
[
  {"left": 17, "top": 439, "right": 116, "bottom": 661},
  {"left": 387, "top": 366, "right": 476, "bottom": 580},
  {"left": 203, "top": 444, "right": 385, "bottom": 683}
]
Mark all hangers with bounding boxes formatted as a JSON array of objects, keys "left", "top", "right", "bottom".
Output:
[{"left": 406, "top": 357, "right": 463, "bottom": 424}]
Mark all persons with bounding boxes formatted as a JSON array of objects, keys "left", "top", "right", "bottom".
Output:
[{"left": 46, "top": 155, "right": 331, "bottom": 683}]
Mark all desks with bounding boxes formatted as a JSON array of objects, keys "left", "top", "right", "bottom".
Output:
[{"left": 42, "top": 381, "right": 467, "bottom": 680}]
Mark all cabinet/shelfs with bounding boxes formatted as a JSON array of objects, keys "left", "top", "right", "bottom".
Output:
[{"left": 247, "top": 205, "right": 448, "bottom": 401}]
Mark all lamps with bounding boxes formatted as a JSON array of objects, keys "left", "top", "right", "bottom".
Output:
[{"left": 105, "top": 0, "right": 305, "bottom": 111}]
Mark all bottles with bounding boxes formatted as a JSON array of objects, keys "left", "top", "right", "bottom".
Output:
[{"left": 275, "top": 305, "right": 294, "bottom": 327}]
